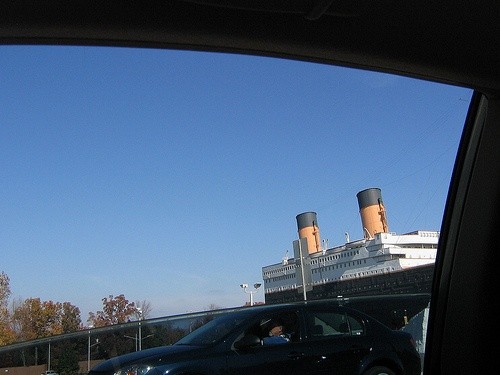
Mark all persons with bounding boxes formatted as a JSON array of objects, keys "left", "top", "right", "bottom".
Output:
[{"left": 268, "top": 319, "right": 289, "bottom": 343}]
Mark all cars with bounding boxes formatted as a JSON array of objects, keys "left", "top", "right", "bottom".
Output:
[{"left": 89, "top": 304, "right": 422, "bottom": 375}]
